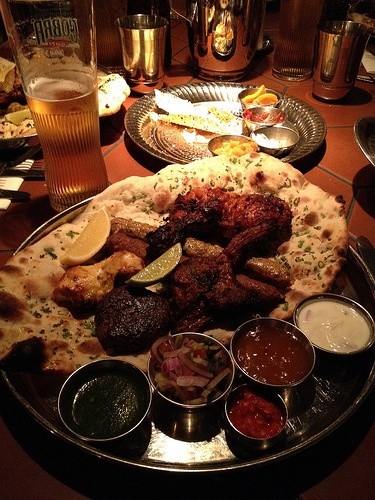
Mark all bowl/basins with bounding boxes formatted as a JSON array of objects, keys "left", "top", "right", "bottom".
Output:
[{"left": 0, "top": 108, "right": 39, "bottom": 147}]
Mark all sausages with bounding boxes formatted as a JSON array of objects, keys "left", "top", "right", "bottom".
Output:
[{"left": 106, "top": 217, "right": 295, "bottom": 305}]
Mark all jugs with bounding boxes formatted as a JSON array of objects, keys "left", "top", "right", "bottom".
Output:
[{"left": 169, "top": 0, "right": 266, "bottom": 83}]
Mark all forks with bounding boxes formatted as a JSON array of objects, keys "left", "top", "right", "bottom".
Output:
[{"left": 0, "top": 143, "right": 42, "bottom": 170}]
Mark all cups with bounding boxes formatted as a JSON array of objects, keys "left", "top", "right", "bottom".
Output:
[
  {"left": 231, "top": 317, "right": 314, "bottom": 390},
  {"left": 113, "top": 15, "right": 170, "bottom": 95},
  {"left": 0, "top": 0, "right": 109, "bottom": 212},
  {"left": 242, "top": 106, "right": 288, "bottom": 132},
  {"left": 238, "top": 87, "right": 282, "bottom": 110},
  {"left": 293, "top": 293, "right": 374, "bottom": 356},
  {"left": 209, "top": 134, "right": 258, "bottom": 157},
  {"left": 272, "top": 0, "right": 325, "bottom": 81},
  {"left": 251, "top": 126, "right": 301, "bottom": 159},
  {"left": 57, "top": 358, "right": 152, "bottom": 443},
  {"left": 147, "top": 331, "right": 235, "bottom": 411},
  {"left": 224, "top": 382, "right": 287, "bottom": 450},
  {"left": 312, "top": 19, "right": 372, "bottom": 100}
]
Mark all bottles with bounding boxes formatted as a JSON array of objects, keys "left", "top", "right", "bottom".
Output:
[{"left": 127, "top": 0, "right": 172, "bottom": 71}]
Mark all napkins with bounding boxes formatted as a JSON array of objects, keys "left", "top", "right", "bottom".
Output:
[
  {"left": 0, "top": 159, "right": 35, "bottom": 211},
  {"left": 361, "top": 49, "right": 375, "bottom": 73}
]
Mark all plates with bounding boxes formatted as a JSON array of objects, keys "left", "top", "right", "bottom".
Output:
[
  {"left": 353, "top": 114, "right": 375, "bottom": 168},
  {"left": 124, "top": 82, "right": 328, "bottom": 164},
  {"left": 0, "top": 208, "right": 375, "bottom": 472}
]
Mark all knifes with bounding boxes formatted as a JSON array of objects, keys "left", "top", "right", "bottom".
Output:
[
  {"left": 357, "top": 234, "right": 375, "bottom": 275},
  {"left": 0, "top": 189, "right": 31, "bottom": 199},
  {"left": 0, "top": 168, "right": 46, "bottom": 177}
]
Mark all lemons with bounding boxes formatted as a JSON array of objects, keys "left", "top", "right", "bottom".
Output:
[
  {"left": 5, "top": 109, "right": 33, "bottom": 126},
  {"left": 125, "top": 243, "right": 183, "bottom": 286},
  {"left": 60, "top": 207, "right": 111, "bottom": 265}
]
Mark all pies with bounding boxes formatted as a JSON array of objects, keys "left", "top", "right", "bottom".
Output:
[
  {"left": 0, "top": 152, "right": 349, "bottom": 379},
  {"left": 0, "top": 58, "right": 130, "bottom": 139}
]
